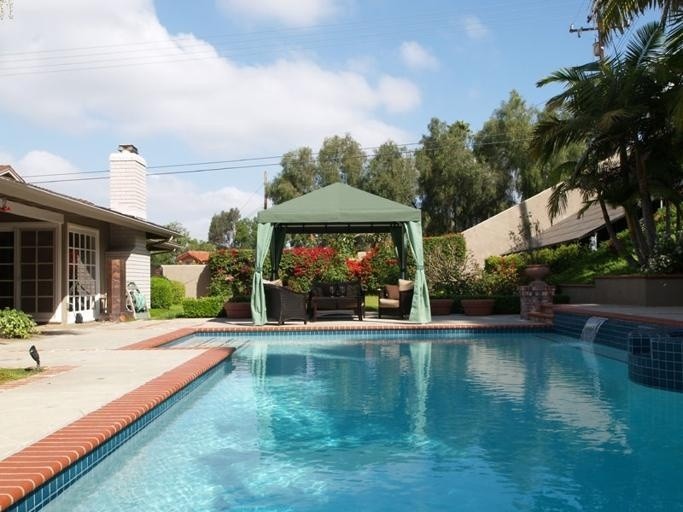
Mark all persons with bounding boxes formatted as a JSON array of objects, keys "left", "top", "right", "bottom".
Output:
[{"left": 589, "top": 232, "right": 598, "bottom": 252}]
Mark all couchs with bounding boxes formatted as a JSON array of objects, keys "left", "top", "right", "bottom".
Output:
[{"left": 309, "top": 281, "right": 366, "bottom": 317}]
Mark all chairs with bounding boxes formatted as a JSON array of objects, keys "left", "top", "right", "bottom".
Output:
[
  {"left": 264, "top": 284, "right": 307, "bottom": 325},
  {"left": 378, "top": 288, "right": 414, "bottom": 319}
]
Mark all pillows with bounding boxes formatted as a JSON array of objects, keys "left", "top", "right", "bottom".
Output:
[
  {"left": 384, "top": 285, "right": 399, "bottom": 298},
  {"left": 399, "top": 279, "right": 413, "bottom": 290},
  {"left": 263, "top": 279, "right": 282, "bottom": 287},
  {"left": 330, "top": 285, "right": 345, "bottom": 297}
]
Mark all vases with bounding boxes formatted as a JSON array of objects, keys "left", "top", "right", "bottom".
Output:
[
  {"left": 524, "top": 264, "right": 549, "bottom": 281},
  {"left": 462, "top": 299, "right": 495, "bottom": 315},
  {"left": 430, "top": 299, "right": 453, "bottom": 314},
  {"left": 224, "top": 302, "right": 251, "bottom": 317}
]
